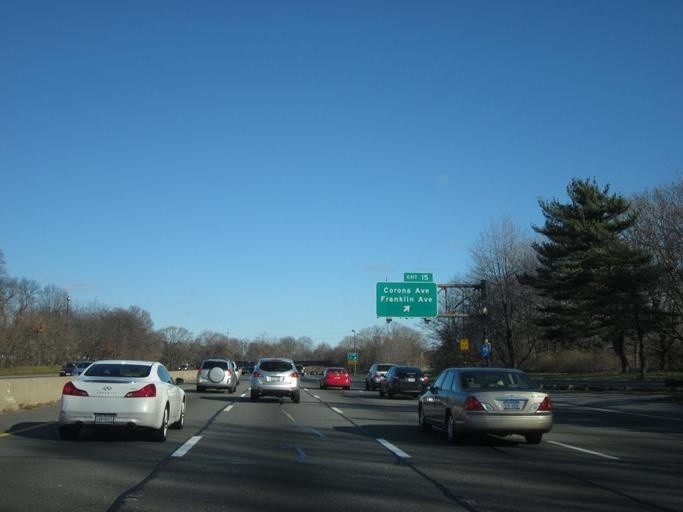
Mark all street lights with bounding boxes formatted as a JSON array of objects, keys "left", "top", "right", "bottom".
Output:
[{"left": 350, "top": 329, "right": 357, "bottom": 376}]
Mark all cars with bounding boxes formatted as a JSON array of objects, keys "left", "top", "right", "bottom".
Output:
[
  {"left": 320, "top": 367, "right": 351, "bottom": 390},
  {"left": 57, "top": 358, "right": 187, "bottom": 444},
  {"left": 415, "top": 366, "right": 556, "bottom": 446},
  {"left": 379, "top": 366, "right": 432, "bottom": 399}
]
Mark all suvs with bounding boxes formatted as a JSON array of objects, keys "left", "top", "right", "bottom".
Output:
[
  {"left": 195, "top": 357, "right": 240, "bottom": 394},
  {"left": 364, "top": 362, "right": 400, "bottom": 392},
  {"left": 247, "top": 356, "right": 301, "bottom": 404}
]
